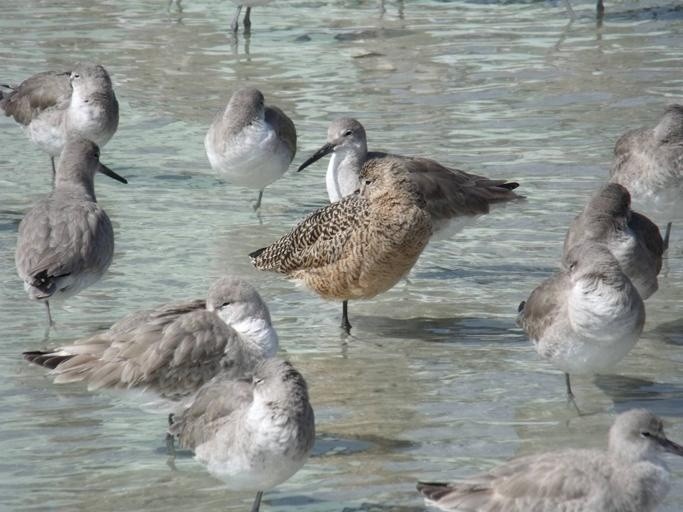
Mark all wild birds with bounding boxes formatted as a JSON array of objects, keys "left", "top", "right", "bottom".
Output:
[
  {"left": 416, "top": 407, "right": 682, "bottom": 512},
  {"left": 245, "top": 156, "right": 434, "bottom": 338},
  {"left": 20, "top": 273, "right": 315, "bottom": 512},
  {"left": 0, "top": 61, "right": 119, "bottom": 172},
  {"left": 610, "top": 103, "right": 683, "bottom": 249},
  {"left": 203, "top": 87, "right": 297, "bottom": 210},
  {"left": 563, "top": 182, "right": 663, "bottom": 300},
  {"left": 515, "top": 239, "right": 647, "bottom": 399},
  {"left": 14, "top": 137, "right": 128, "bottom": 324},
  {"left": 296, "top": 115, "right": 526, "bottom": 234}
]
